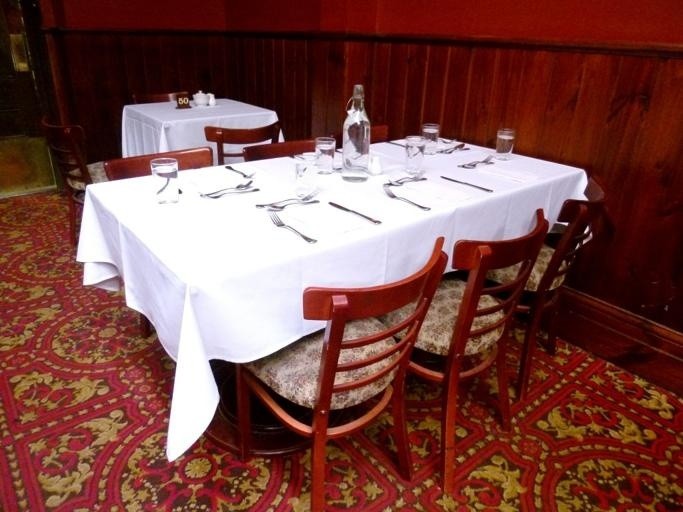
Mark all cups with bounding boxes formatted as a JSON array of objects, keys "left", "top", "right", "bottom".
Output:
[
  {"left": 421, "top": 123, "right": 440, "bottom": 155},
  {"left": 315, "top": 136, "right": 336, "bottom": 176},
  {"left": 495, "top": 127, "right": 515, "bottom": 160},
  {"left": 405, "top": 136, "right": 425, "bottom": 170},
  {"left": 150, "top": 157, "right": 180, "bottom": 205}
]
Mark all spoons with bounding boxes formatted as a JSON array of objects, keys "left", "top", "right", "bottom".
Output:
[
  {"left": 437, "top": 143, "right": 465, "bottom": 154},
  {"left": 463, "top": 162, "right": 495, "bottom": 169},
  {"left": 200, "top": 180, "right": 253, "bottom": 197},
  {"left": 389, "top": 177, "right": 428, "bottom": 186},
  {"left": 264, "top": 200, "right": 320, "bottom": 211}
]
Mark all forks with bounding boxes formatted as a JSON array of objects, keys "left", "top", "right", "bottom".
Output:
[
  {"left": 268, "top": 211, "right": 317, "bottom": 244},
  {"left": 225, "top": 165, "right": 257, "bottom": 178},
  {"left": 457, "top": 154, "right": 493, "bottom": 168},
  {"left": 255, "top": 189, "right": 321, "bottom": 209},
  {"left": 384, "top": 188, "right": 431, "bottom": 211}
]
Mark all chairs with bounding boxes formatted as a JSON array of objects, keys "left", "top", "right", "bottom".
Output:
[
  {"left": 236, "top": 235, "right": 449, "bottom": 512},
  {"left": 484, "top": 177, "right": 606, "bottom": 401},
  {"left": 370, "top": 125, "right": 389, "bottom": 144},
  {"left": 104, "top": 146, "right": 212, "bottom": 339},
  {"left": 131, "top": 91, "right": 189, "bottom": 104},
  {"left": 204, "top": 119, "right": 281, "bottom": 165},
  {"left": 39, "top": 114, "right": 109, "bottom": 249},
  {"left": 243, "top": 139, "right": 315, "bottom": 162},
  {"left": 380, "top": 208, "right": 548, "bottom": 432}
]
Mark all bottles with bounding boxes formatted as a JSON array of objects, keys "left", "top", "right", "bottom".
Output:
[
  {"left": 341, "top": 84, "right": 371, "bottom": 185},
  {"left": 206, "top": 93, "right": 216, "bottom": 107}
]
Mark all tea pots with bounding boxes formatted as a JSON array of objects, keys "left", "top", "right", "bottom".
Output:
[{"left": 193, "top": 90, "right": 210, "bottom": 107}]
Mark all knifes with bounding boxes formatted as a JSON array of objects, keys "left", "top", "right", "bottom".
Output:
[
  {"left": 328, "top": 201, "right": 382, "bottom": 224},
  {"left": 440, "top": 175, "right": 494, "bottom": 193}
]
